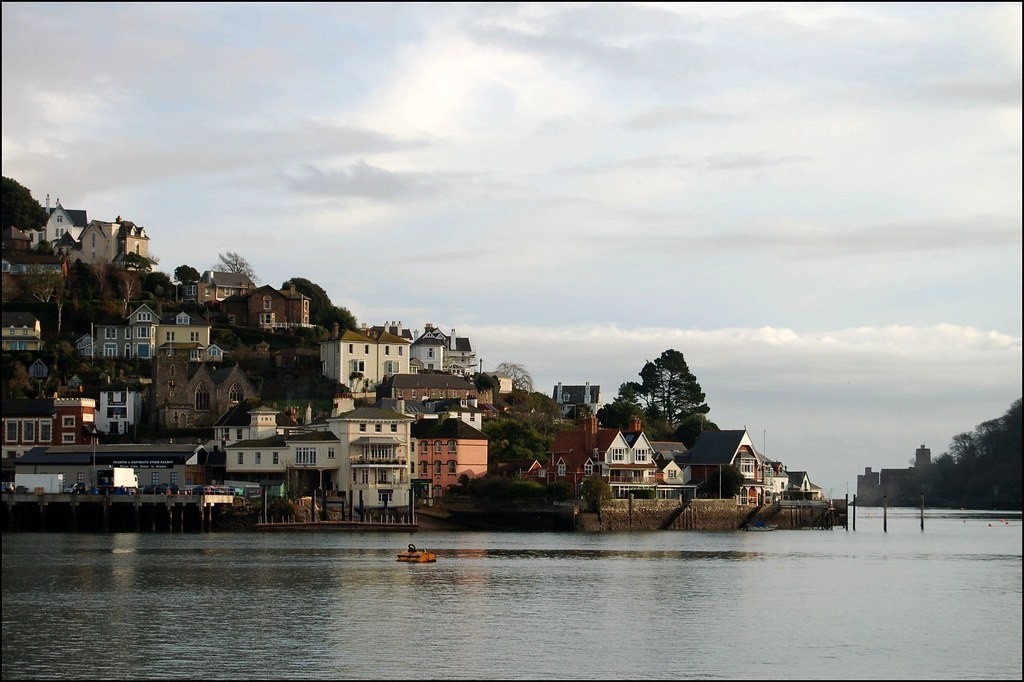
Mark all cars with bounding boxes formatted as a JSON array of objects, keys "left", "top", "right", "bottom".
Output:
[
  {"left": 63, "top": 483, "right": 92, "bottom": 495},
  {"left": 144, "top": 482, "right": 180, "bottom": 496},
  {"left": 187, "top": 484, "right": 226, "bottom": 496}
]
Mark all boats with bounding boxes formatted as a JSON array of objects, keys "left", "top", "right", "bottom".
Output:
[{"left": 396, "top": 544, "right": 437, "bottom": 563}]
